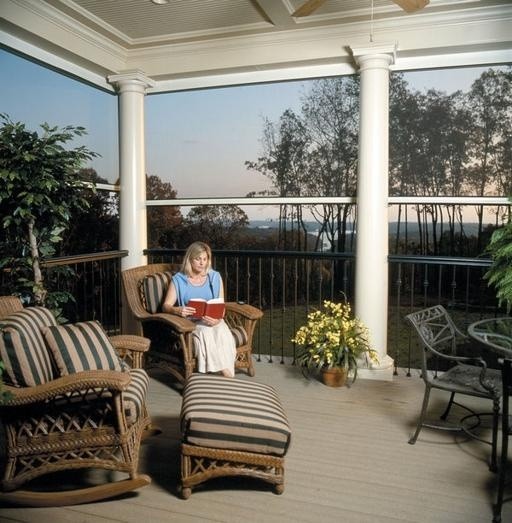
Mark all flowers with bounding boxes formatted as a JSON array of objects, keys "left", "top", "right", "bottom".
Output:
[{"left": 291, "top": 291, "right": 379, "bottom": 386}]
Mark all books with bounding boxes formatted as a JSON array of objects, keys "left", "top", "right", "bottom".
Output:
[{"left": 186, "top": 298, "right": 227, "bottom": 323}]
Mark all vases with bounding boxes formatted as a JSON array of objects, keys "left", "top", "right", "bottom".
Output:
[{"left": 322, "top": 364, "right": 346, "bottom": 386}]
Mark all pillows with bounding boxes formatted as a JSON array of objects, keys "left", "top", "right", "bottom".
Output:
[
  {"left": 43, "top": 323, "right": 122, "bottom": 393},
  {"left": 0, "top": 306, "right": 54, "bottom": 390}
]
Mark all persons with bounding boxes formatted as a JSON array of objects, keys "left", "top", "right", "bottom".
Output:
[{"left": 162, "top": 242, "right": 237, "bottom": 379}]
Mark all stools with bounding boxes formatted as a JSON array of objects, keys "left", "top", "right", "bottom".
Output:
[{"left": 178, "top": 371, "right": 290, "bottom": 499}]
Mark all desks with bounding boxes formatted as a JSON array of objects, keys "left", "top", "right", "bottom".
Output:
[{"left": 468, "top": 318, "right": 512, "bottom": 523}]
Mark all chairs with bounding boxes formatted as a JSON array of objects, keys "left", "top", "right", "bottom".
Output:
[
  {"left": 406, "top": 305, "right": 503, "bottom": 473},
  {"left": 0, "top": 297, "right": 162, "bottom": 507},
  {"left": 123, "top": 263, "right": 263, "bottom": 388}
]
